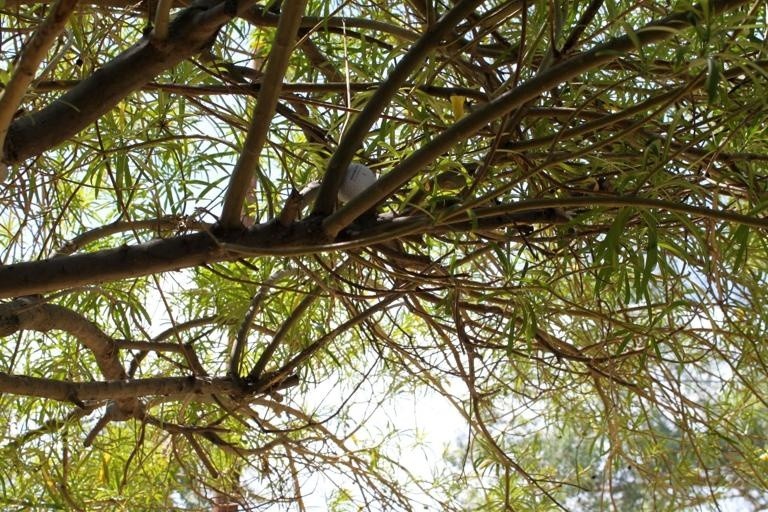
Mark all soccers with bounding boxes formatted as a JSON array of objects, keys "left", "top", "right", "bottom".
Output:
[{"left": 338, "top": 163, "right": 375, "bottom": 201}]
